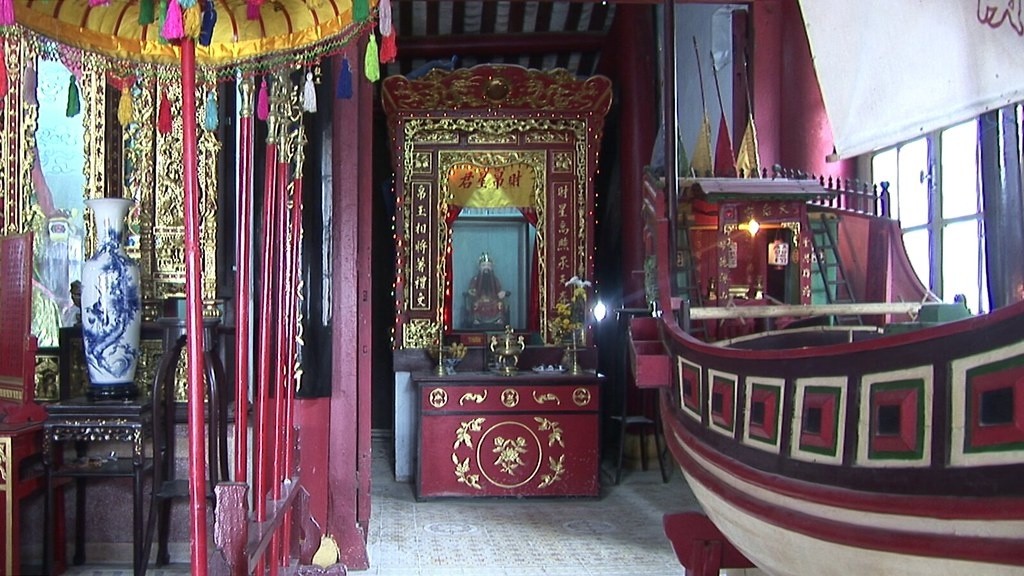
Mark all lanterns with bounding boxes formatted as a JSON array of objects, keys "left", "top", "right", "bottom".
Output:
[{"left": 768, "top": 240, "right": 789, "bottom": 270}]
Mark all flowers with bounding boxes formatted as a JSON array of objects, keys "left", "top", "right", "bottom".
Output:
[{"left": 553, "top": 276, "right": 592, "bottom": 338}]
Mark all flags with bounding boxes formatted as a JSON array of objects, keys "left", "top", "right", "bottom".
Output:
[
  {"left": 736, "top": 119, "right": 760, "bottom": 179},
  {"left": 678, "top": 138, "right": 689, "bottom": 177},
  {"left": 714, "top": 114, "right": 738, "bottom": 178},
  {"left": 690, "top": 121, "right": 713, "bottom": 178}
]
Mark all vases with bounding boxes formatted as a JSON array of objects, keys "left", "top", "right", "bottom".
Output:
[
  {"left": 561, "top": 339, "right": 574, "bottom": 368},
  {"left": 80, "top": 198, "right": 142, "bottom": 401}
]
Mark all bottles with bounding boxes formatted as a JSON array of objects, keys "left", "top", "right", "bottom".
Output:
[{"left": 80, "top": 198, "right": 140, "bottom": 400}]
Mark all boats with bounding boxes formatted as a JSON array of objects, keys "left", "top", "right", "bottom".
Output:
[{"left": 652, "top": 289, "right": 1024, "bottom": 576}]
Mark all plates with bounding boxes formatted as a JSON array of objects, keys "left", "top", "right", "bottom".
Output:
[{"left": 534, "top": 370, "right": 567, "bottom": 375}]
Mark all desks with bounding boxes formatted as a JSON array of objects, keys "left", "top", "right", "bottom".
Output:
[
  {"left": 138, "top": 318, "right": 229, "bottom": 575},
  {"left": 411, "top": 368, "right": 606, "bottom": 503},
  {"left": 41, "top": 395, "right": 170, "bottom": 576},
  {"left": 598, "top": 309, "right": 668, "bottom": 485},
  {"left": 0, "top": 421, "right": 65, "bottom": 576}
]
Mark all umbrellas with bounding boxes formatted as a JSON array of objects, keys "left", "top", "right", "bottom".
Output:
[{"left": 0, "top": 0, "right": 395, "bottom": 576}]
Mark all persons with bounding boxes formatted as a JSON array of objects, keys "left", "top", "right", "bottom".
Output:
[{"left": 468, "top": 253, "right": 511, "bottom": 325}]
